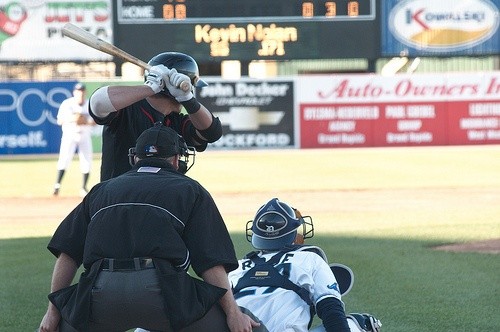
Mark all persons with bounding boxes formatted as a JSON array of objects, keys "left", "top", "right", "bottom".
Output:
[
  {"left": 36, "top": 122, "right": 268, "bottom": 332},
  {"left": 88, "top": 52, "right": 222, "bottom": 183},
  {"left": 54, "top": 82, "right": 98, "bottom": 196},
  {"left": 226, "top": 199, "right": 382, "bottom": 332}
]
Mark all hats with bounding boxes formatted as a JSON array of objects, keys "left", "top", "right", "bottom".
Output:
[{"left": 74, "top": 82, "right": 86, "bottom": 91}]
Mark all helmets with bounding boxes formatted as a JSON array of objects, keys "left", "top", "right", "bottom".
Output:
[
  {"left": 251, "top": 198, "right": 303, "bottom": 251},
  {"left": 145, "top": 52, "right": 208, "bottom": 88},
  {"left": 134, "top": 123, "right": 187, "bottom": 159}
]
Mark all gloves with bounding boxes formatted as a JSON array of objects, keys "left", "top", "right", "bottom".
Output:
[
  {"left": 164, "top": 66, "right": 194, "bottom": 102},
  {"left": 144, "top": 64, "right": 171, "bottom": 95}
]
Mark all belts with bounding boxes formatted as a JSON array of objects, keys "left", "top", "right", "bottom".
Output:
[{"left": 99, "top": 259, "right": 155, "bottom": 270}]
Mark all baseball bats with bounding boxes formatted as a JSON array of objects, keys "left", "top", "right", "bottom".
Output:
[{"left": 62, "top": 22, "right": 190, "bottom": 92}]
